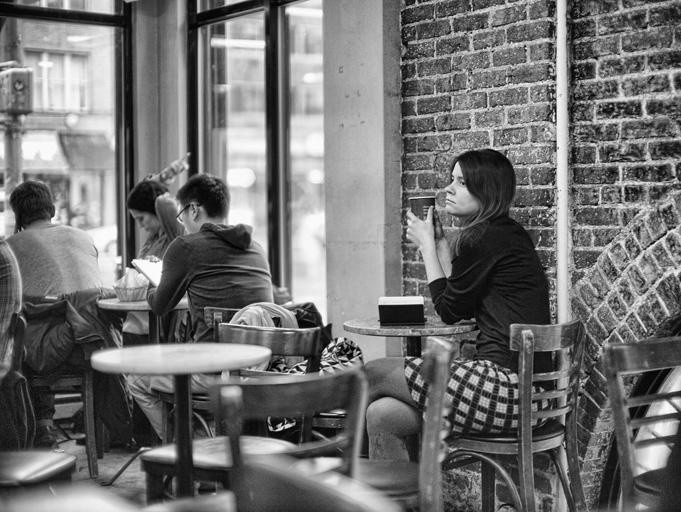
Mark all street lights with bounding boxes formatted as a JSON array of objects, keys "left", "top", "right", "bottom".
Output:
[{"left": 266, "top": 338, "right": 364, "bottom": 433}]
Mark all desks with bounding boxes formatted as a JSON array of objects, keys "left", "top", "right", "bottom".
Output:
[{"left": 344, "top": 316, "right": 480, "bottom": 356}]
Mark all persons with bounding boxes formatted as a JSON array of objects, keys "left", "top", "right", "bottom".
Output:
[
  {"left": 122, "top": 150, "right": 192, "bottom": 344},
  {"left": 1, "top": 235, "right": 24, "bottom": 380},
  {"left": 123, "top": 171, "right": 275, "bottom": 492},
  {"left": 6, "top": 181, "right": 105, "bottom": 447},
  {"left": 362, "top": 148, "right": 555, "bottom": 461}
]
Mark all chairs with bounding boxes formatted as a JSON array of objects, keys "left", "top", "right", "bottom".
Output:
[
  {"left": 443, "top": 318, "right": 579, "bottom": 510},
  {"left": 1, "top": 288, "right": 457, "bottom": 511},
  {"left": 33, "top": 424, "right": 58, "bottom": 450},
  {"left": 602, "top": 336, "right": 679, "bottom": 510}
]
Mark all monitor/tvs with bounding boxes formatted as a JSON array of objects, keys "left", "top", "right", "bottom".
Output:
[{"left": 130, "top": 259, "right": 162, "bottom": 288}]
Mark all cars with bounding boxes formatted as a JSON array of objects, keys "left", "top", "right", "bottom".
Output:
[{"left": 408, "top": 196, "right": 436, "bottom": 220}]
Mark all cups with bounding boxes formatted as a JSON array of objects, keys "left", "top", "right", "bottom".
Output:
[{"left": 176, "top": 203, "right": 201, "bottom": 223}]
